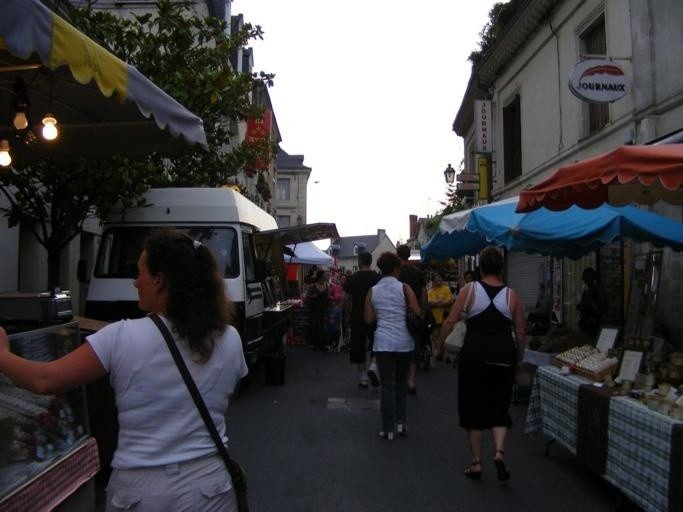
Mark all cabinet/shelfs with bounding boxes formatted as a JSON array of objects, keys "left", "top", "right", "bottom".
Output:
[
  {"left": 602, "top": 386, "right": 683, "bottom": 512},
  {"left": 0, "top": 319, "right": 102, "bottom": 512},
  {"left": 521, "top": 361, "right": 615, "bottom": 471}
]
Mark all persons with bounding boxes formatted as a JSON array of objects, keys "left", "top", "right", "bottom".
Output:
[
  {"left": 0, "top": 233, "right": 249, "bottom": 512},
  {"left": 574, "top": 266, "right": 603, "bottom": 335},
  {"left": 305, "top": 269, "right": 352, "bottom": 354},
  {"left": 394, "top": 244, "right": 429, "bottom": 394},
  {"left": 342, "top": 251, "right": 383, "bottom": 388},
  {"left": 427, "top": 273, "right": 453, "bottom": 364},
  {"left": 456, "top": 270, "right": 475, "bottom": 295},
  {"left": 363, "top": 250, "right": 425, "bottom": 441},
  {"left": 433, "top": 246, "right": 525, "bottom": 480}
]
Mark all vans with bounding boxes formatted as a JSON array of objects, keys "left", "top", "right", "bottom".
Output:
[{"left": 84, "top": 186, "right": 340, "bottom": 388}]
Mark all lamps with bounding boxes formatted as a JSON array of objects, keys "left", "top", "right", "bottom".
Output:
[
  {"left": 41, "top": 111, "right": 58, "bottom": 142},
  {"left": 1, "top": 136, "right": 13, "bottom": 169},
  {"left": 14, "top": 100, "right": 29, "bottom": 130}
]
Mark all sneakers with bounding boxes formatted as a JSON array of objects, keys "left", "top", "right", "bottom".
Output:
[
  {"left": 395, "top": 424, "right": 409, "bottom": 434},
  {"left": 377, "top": 431, "right": 391, "bottom": 439},
  {"left": 367, "top": 364, "right": 378, "bottom": 386},
  {"left": 356, "top": 377, "right": 369, "bottom": 389}
]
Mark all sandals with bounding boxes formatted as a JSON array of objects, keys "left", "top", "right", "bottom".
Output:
[
  {"left": 492, "top": 449, "right": 511, "bottom": 485},
  {"left": 459, "top": 461, "right": 483, "bottom": 479}
]
